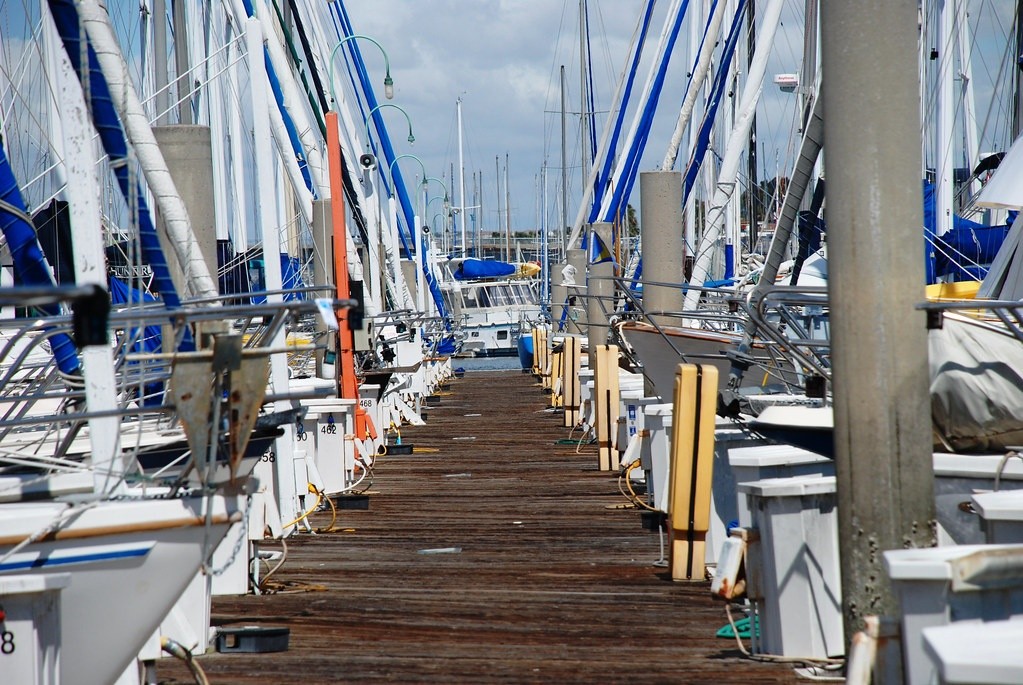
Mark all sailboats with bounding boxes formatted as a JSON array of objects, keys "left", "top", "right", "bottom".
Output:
[{"left": 0, "top": 0, "right": 1023, "bottom": 685}]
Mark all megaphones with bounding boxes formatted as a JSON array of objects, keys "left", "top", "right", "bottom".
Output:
[
  {"left": 360, "top": 154, "right": 376, "bottom": 170},
  {"left": 422, "top": 226, "right": 430, "bottom": 235}
]
